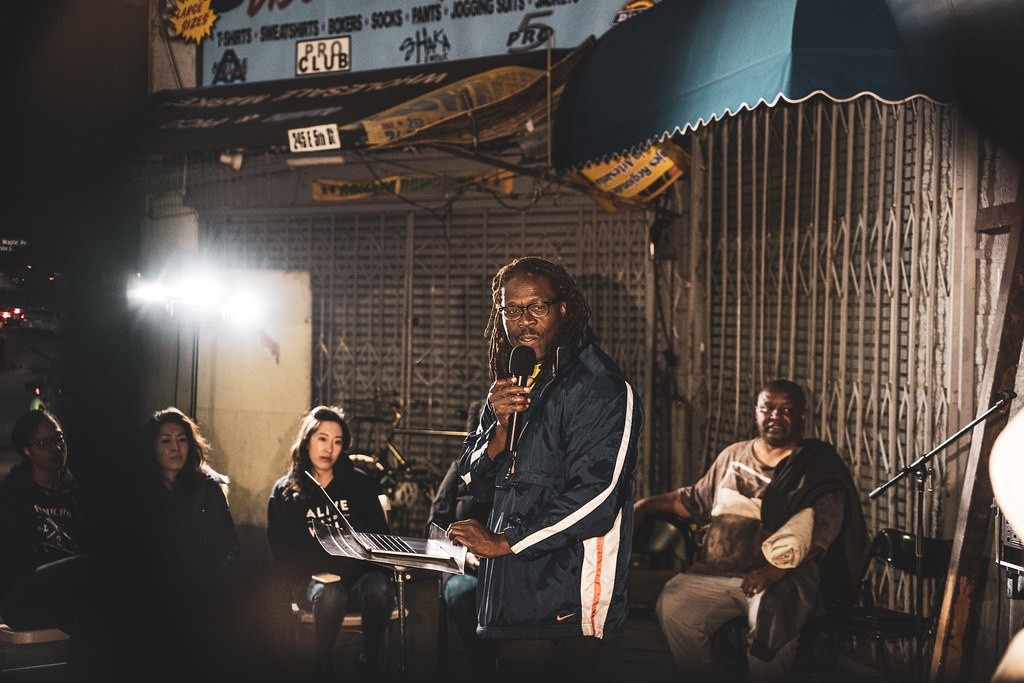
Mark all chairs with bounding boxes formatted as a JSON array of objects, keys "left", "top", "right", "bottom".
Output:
[
  {"left": 608, "top": 515, "right": 695, "bottom": 683},
  {"left": 796, "top": 527, "right": 955, "bottom": 683},
  {"left": 288, "top": 591, "right": 409, "bottom": 683}
]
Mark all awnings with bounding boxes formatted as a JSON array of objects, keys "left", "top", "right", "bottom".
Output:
[{"left": 552, "top": 0, "right": 950, "bottom": 169}]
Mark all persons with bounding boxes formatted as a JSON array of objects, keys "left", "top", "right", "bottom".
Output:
[
  {"left": 0, "top": 411, "right": 85, "bottom": 636},
  {"left": 138, "top": 408, "right": 240, "bottom": 585},
  {"left": 634, "top": 380, "right": 870, "bottom": 683},
  {"left": 267, "top": 405, "right": 395, "bottom": 682},
  {"left": 444, "top": 257, "right": 645, "bottom": 683},
  {"left": 424, "top": 400, "right": 495, "bottom": 682}
]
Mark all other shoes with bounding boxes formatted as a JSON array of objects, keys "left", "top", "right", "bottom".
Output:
[
  {"left": 358, "top": 655, "right": 384, "bottom": 682},
  {"left": 312, "top": 659, "right": 333, "bottom": 683}
]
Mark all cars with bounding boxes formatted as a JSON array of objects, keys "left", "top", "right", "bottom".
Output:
[{"left": 19, "top": 308, "right": 67, "bottom": 338}]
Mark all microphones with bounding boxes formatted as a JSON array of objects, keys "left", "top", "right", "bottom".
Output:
[
  {"left": 379, "top": 412, "right": 402, "bottom": 455},
  {"left": 509, "top": 345, "right": 536, "bottom": 452}
]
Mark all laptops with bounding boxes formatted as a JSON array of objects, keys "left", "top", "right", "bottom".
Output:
[{"left": 305, "top": 471, "right": 450, "bottom": 561}]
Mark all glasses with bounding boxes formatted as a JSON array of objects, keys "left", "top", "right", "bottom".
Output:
[
  {"left": 28, "top": 433, "right": 65, "bottom": 449},
  {"left": 499, "top": 296, "right": 564, "bottom": 321}
]
